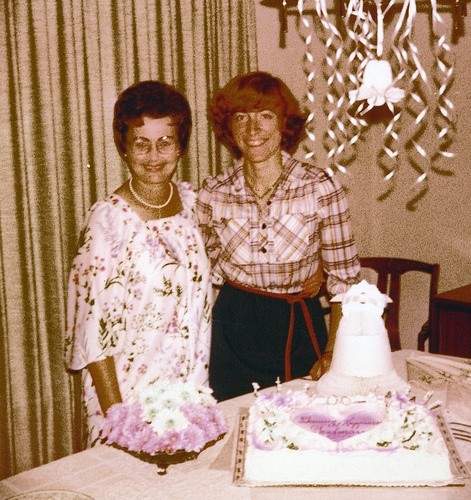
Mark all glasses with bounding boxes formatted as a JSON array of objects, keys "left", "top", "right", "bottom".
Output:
[{"left": 124, "top": 133, "right": 178, "bottom": 154}]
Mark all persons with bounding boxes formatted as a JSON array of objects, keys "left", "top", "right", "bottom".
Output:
[
  {"left": 65, "top": 81, "right": 213, "bottom": 450},
  {"left": 196, "top": 72, "right": 360, "bottom": 402}
]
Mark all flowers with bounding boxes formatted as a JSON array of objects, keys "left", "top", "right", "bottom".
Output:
[{"left": 99, "top": 372, "right": 228, "bottom": 475}]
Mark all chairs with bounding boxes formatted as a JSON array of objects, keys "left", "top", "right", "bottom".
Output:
[{"left": 358, "top": 258, "right": 441, "bottom": 353}]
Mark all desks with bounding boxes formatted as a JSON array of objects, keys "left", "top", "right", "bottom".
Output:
[
  {"left": 431, "top": 285, "right": 471, "bottom": 359},
  {"left": 0, "top": 347, "right": 471, "bottom": 499}
]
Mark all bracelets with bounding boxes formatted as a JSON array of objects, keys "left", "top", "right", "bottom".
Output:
[{"left": 323, "top": 350, "right": 333, "bottom": 353}]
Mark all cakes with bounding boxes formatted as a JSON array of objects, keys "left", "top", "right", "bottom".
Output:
[{"left": 241, "top": 390, "right": 455, "bottom": 485}]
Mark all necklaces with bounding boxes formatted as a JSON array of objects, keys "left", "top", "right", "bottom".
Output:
[
  {"left": 129, "top": 177, "right": 174, "bottom": 209},
  {"left": 247, "top": 174, "right": 282, "bottom": 207}
]
